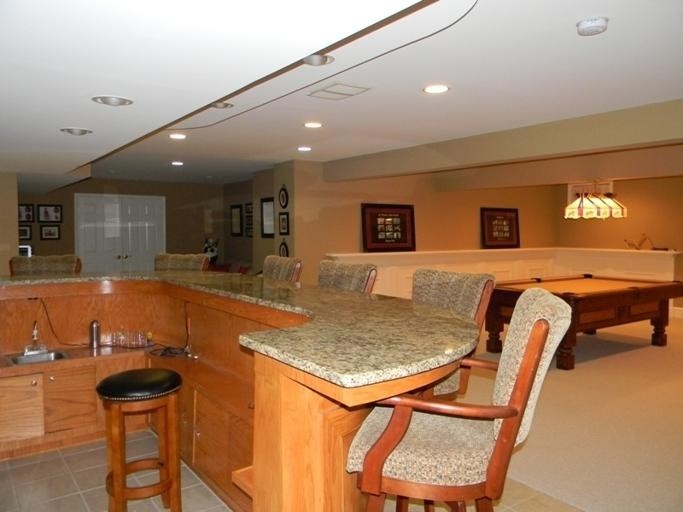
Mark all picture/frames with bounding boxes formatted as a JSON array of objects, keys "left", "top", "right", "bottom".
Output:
[
  {"left": 17, "top": 202, "right": 63, "bottom": 242},
  {"left": 359, "top": 201, "right": 417, "bottom": 252},
  {"left": 228, "top": 184, "right": 290, "bottom": 257},
  {"left": 478, "top": 206, "right": 521, "bottom": 249}
]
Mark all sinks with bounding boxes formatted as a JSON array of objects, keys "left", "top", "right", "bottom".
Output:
[{"left": 8, "top": 350, "right": 67, "bottom": 366}]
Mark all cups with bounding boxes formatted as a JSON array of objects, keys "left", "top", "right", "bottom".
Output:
[{"left": 111, "top": 330, "right": 152, "bottom": 346}]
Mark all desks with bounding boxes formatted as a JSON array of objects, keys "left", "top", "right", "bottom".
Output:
[
  {"left": 208, "top": 262, "right": 232, "bottom": 272},
  {"left": 481, "top": 272, "right": 682, "bottom": 372}
]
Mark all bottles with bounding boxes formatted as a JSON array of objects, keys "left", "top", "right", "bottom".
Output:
[
  {"left": 88, "top": 347, "right": 99, "bottom": 358},
  {"left": 89, "top": 320, "right": 100, "bottom": 348}
]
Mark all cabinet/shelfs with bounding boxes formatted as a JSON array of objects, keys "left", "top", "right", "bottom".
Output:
[
  {"left": 145, "top": 349, "right": 170, "bottom": 443},
  {"left": 1, "top": 340, "right": 95, "bottom": 465},
  {"left": 93, "top": 340, "right": 153, "bottom": 443},
  {"left": 164, "top": 348, "right": 226, "bottom": 507},
  {"left": 223, "top": 374, "right": 255, "bottom": 512}
]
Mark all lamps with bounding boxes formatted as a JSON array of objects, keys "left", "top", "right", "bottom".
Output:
[
  {"left": 561, "top": 178, "right": 629, "bottom": 222},
  {"left": 192, "top": 225, "right": 225, "bottom": 262}
]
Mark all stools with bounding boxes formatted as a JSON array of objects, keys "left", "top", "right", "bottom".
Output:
[{"left": 93, "top": 367, "right": 186, "bottom": 512}]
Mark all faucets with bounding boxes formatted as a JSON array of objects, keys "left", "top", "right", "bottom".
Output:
[{"left": 31, "top": 320, "right": 40, "bottom": 349}]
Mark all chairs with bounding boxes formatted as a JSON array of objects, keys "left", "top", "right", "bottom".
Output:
[
  {"left": 406, "top": 266, "right": 497, "bottom": 407},
  {"left": 315, "top": 257, "right": 379, "bottom": 295},
  {"left": 344, "top": 285, "right": 576, "bottom": 511},
  {"left": 236, "top": 259, "right": 257, "bottom": 275},
  {"left": 259, "top": 255, "right": 305, "bottom": 283},
  {"left": 149, "top": 248, "right": 210, "bottom": 273},
  {"left": 7, "top": 252, "right": 82, "bottom": 279}
]
